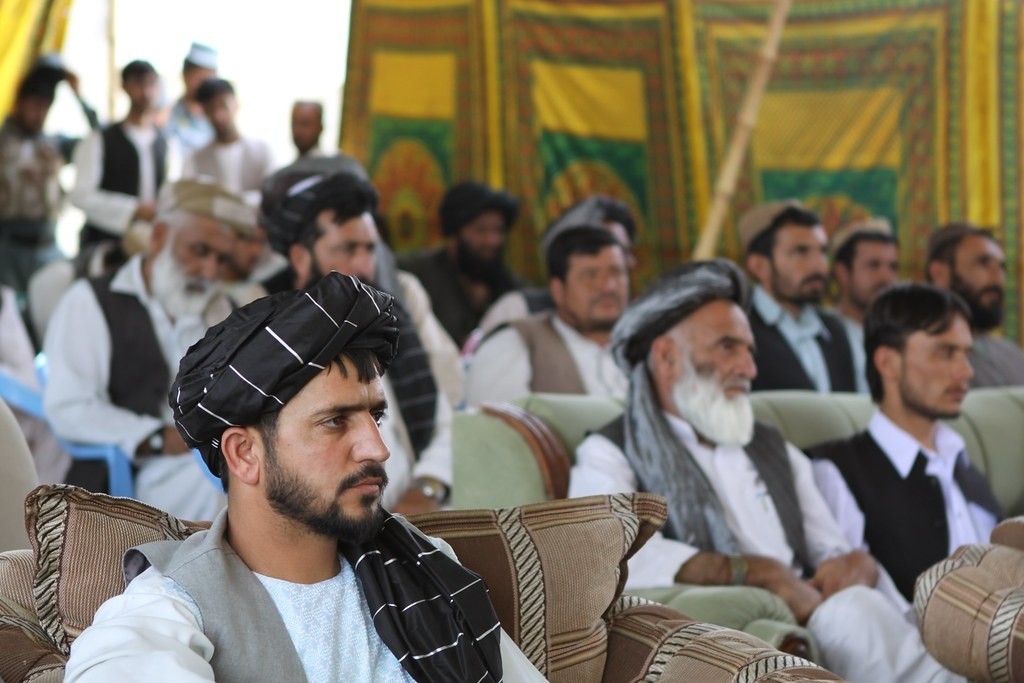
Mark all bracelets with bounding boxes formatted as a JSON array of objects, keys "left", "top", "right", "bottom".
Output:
[
  {"left": 407, "top": 483, "right": 443, "bottom": 502},
  {"left": 62, "top": 272, "right": 503, "bottom": 683},
  {"left": 148, "top": 427, "right": 168, "bottom": 453}
]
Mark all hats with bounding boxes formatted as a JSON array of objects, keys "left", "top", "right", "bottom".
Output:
[
  {"left": 185, "top": 43, "right": 220, "bottom": 68},
  {"left": 828, "top": 217, "right": 892, "bottom": 260},
  {"left": 160, "top": 178, "right": 257, "bottom": 237},
  {"left": 440, "top": 181, "right": 523, "bottom": 239},
  {"left": 738, "top": 198, "right": 803, "bottom": 250}
]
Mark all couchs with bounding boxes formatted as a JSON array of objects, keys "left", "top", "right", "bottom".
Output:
[
  {"left": 914, "top": 515, "right": 1023, "bottom": 681},
  {"left": 453, "top": 387, "right": 1024, "bottom": 669},
  {"left": 1, "top": 547, "right": 838, "bottom": 683}
]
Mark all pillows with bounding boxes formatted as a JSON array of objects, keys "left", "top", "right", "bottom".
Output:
[{"left": 27, "top": 482, "right": 665, "bottom": 683}]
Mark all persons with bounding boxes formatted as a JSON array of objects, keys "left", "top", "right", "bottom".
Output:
[
  {"left": 736, "top": 199, "right": 872, "bottom": 392},
  {"left": 568, "top": 256, "right": 966, "bottom": 682},
  {"left": 0, "top": 40, "right": 463, "bottom": 523},
  {"left": 921, "top": 222, "right": 1024, "bottom": 392},
  {"left": 394, "top": 177, "right": 532, "bottom": 352},
  {"left": 800, "top": 281, "right": 1004, "bottom": 624},
  {"left": 832, "top": 214, "right": 900, "bottom": 320},
  {"left": 460, "top": 194, "right": 638, "bottom": 408}
]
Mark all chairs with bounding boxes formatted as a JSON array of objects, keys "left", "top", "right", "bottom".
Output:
[{"left": 31, "top": 352, "right": 139, "bottom": 496}]
